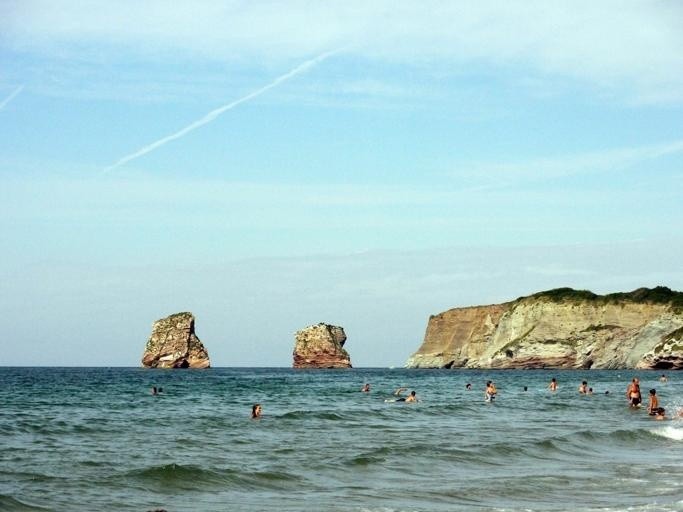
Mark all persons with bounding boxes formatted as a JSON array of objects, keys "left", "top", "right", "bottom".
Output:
[
  {"left": 384, "top": 391, "right": 416, "bottom": 403},
  {"left": 483, "top": 380, "right": 495, "bottom": 403},
  {"left": 584, "top": 387, "right": 592, "bottom": 395},
  {"left": 578, "top": 381, "right": 586, "bottom": 394},
  {"left": 648, "top": 389, "right": 658, "bottom": 416},
  {"left": 626, "top": 377, "right": 641, "bottom": 406},
  {"left": 361, "top": 384, "right": 369, "bottom": 392},
  {"left": 659, "top": 374, "right": 667, "bottom": 382},
  {"left": 655, "top": 407, "right": 665, "bottom": 419},
  {"left": 250, "top": 403, "right": 261, "bottom": 420},
  {"left": 546, "top": 378, "right": 557, "bottom": 392}
]
